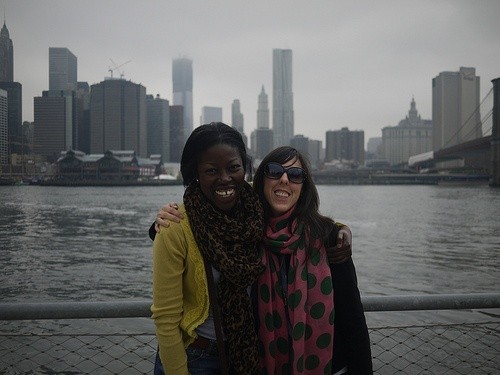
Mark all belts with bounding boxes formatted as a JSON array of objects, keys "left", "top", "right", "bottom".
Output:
[{"left": 190, "top": 336, "right": 219, "bottom": 354}]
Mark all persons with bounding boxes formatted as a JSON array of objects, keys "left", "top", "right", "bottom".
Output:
[
  {"left": 152, "top": 120, "right": 353, "bottom": 375},
  {"left": 148, "top": 145, "right": 373, "bottom": 375}
]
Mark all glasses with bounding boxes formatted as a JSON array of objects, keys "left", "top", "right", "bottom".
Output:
[{"left": 264, "top": 162, "right": 309, "bottom": 185}]
